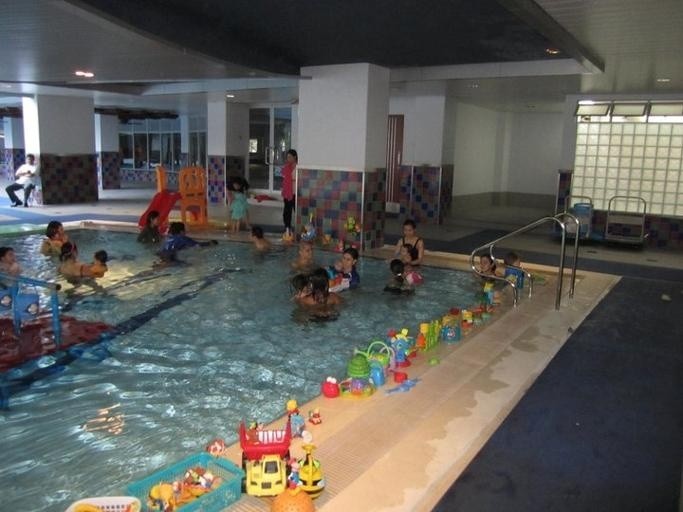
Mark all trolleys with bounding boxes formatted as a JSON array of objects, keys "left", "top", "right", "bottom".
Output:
[
  {"left": 563, "top": 194, "right": 603, "bottom": 243},
  {"left": 604, "top": 194, "right": 649, "bottom": 245}
]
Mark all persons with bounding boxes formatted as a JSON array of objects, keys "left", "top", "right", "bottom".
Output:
[
  {"left": 281, "top": 149, "right": 298, "bottom": 236},
  {"left": 384, "top": 219, "right": 424, "bottom": 298},
  {"left": 39, "top": 219, "right": 107, "bottom": 279},
  {"left": 231, "top": 192, "right": 249, "bottom": 233},
  {"left": 251, "top": 226, "right": 272, "bottom": 252},
  {"left": 5, "top": 157, "right": 36, "bottom": 208},
  {"left": 137, "top": 211, "right": 163, "bottom": 245},
  {"left": 290, "top": 241, "right": 361, "bottom": 320},
  {"left": 225, "top": 176, "right": 252, "bottom": 231},
  {"left": 0, "top": 246, "right": 24, "bottom": 285},
  {"left": 159, "top": 222, "right": 217, "bottom": 260},
  {"left": 477, "top": 254, "right": 499, "bottom": 284},
  {"left": 505, "top": 252, "right": 524, "bottom": 290}
]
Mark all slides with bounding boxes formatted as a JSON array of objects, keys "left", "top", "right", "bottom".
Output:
[{"left": 138, "top": 192, "right": 181, "bottom": 227}]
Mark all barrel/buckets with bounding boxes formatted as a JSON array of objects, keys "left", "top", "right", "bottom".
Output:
[{"left": 574, "top": 203, "right": 594, "bottom": 233}]
